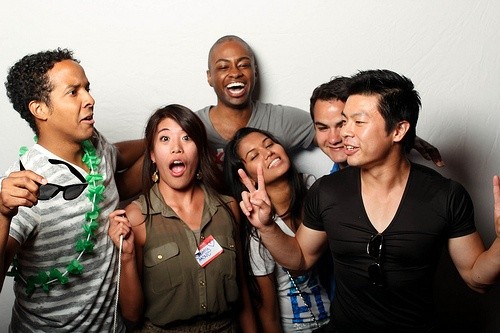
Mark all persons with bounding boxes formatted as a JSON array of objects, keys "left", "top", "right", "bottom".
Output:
[
  {"left": 0, "top": 47, "right": 152, "bottom": 333},
  {"left": 118, "top": 36, "right": 445, "bottom": 333},
  {"left": 108, "top": 104, "right": 258, "bottom": 333},
  {"left": 238, "top": 69, "right": 500, "bottom": 333}
]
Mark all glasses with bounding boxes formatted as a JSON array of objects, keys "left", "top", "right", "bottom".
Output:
[
  {"left": 18, "top": 158, "right": 88, "bottom": 201},
  {"left": 365, "top": 233, "right": 387, "bottom": 289}
]
